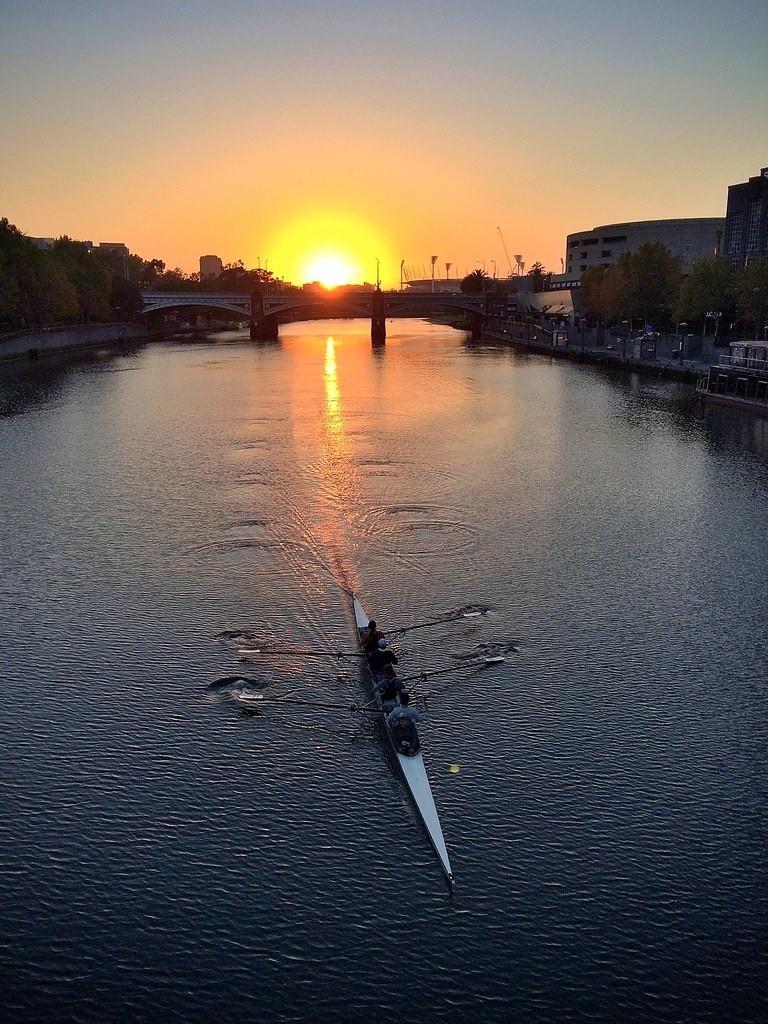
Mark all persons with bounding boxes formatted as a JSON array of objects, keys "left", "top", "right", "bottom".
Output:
[
  {"left": 368, "top": 638, "right": 398, "bottom": 673},
  {"left": 387, "top": 693, "right": 422, "bottom": 728},
  {"left": 393, "top": 713, "right": 418, "bottom": 754},
  {"left": 372, "top": 664, "right": 406, "bottom": 700},
  {"left": 360, "top": 620, "right": 385, "bottom": 646}
]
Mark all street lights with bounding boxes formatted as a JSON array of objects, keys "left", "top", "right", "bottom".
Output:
[
  {"left": 445, "top": 262, "right": 453, "bottom": 279},
  {"left": 400, "top": 260, "right": 405, "bottom": 293},
  {"left": 491, "top": 259, "right": 497, "bottom": 292},
  {"left": 265, "top": 259, "right": 269, "bottom": 270},
  {"left": 477, "top": 260, "right": 486, "bottom": 274},
  {"left": 519, "top": 261, "right": 526, "bottom": 277},
  {"left": 258, "top": 256, "right": 261, "bottom": 270},
  {"left": 430, "top": 256, "right": 440, "bottom": 293},
  {"left": 513, "top": 253, "right": 523, "bottom": 277}
]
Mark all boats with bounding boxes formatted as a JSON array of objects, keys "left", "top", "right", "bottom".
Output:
[
  {"left": 693, "top": 339, "right": 768, "bottom": 416},
  {"left": 351, "top": 591, "right": 458, "bottom": 888}
]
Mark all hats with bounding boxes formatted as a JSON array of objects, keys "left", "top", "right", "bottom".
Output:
[
  {"left": 368, "top": 621, "right": 376, "bottom": 629},
  {"left": 378, "top": 638, "right": 386, "bottom": 647}
]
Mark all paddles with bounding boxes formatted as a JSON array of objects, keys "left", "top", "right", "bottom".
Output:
[
  {"left": 401, "top": 656, "right": 506, "bottom": 680},
  {"left": 238, "top": 649, "right": 366, "bottom": 656},
  {"left": 382, "top": 612, "right": 482, "bottom": 635},
  {"left": 238, "top": 693, "right": 383, "bottom": 712}
]
